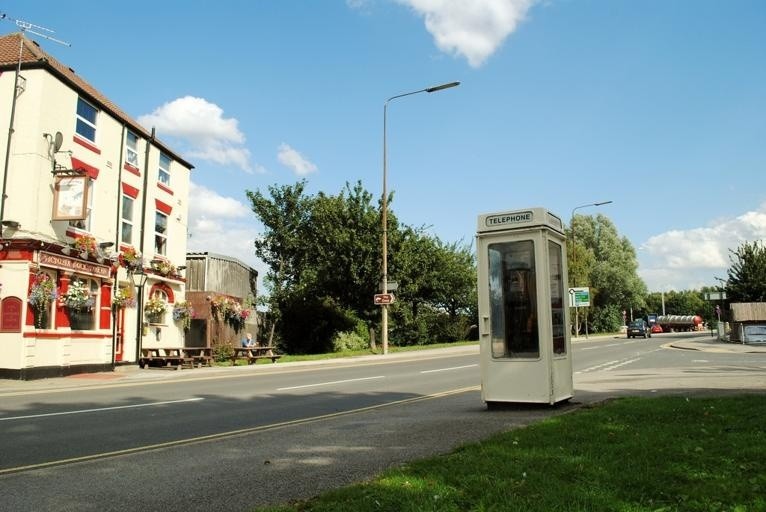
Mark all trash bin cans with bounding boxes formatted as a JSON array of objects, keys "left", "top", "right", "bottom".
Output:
[
  {"left": 620, "top": 326, "right": 629, "bottom": 334},
  {"left": 717, "top": 321, "right": 725, "bottom": 341}
]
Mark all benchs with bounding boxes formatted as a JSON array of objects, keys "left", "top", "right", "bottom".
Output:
[
  {"left": 224, "top": 355, "right": 258, "bottom": 366},
  {"left": 248, "top": 355, "right": 282, "bottom": 365}
]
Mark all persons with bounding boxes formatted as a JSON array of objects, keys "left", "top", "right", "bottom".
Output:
[{"left": 242, "top": 333, "right": 258, "bottom": 360}]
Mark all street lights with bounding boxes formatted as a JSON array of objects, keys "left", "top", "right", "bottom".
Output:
[
  {"left": 572, "top": 201, "right": 611, "bottom": 338},
  {"left": 381, "top": 81, "right": 462, "bottom": 351},
  {"left": 133, "top": 265, "right": 147, "bottom": 365}
]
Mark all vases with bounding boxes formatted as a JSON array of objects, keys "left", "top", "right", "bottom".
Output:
[
  {"left": 149, "top": 313, "right": 162, "bottom": 323},
  {"left": 34, "top": 309, "right": 47, "bottom": 329},
  {"left": 70, "top": 310, "right": 91, "bottom": 330}
]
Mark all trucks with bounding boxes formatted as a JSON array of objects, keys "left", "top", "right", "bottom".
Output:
[{"left": 643, "top": 314, "right": 704, "bottom": 332}]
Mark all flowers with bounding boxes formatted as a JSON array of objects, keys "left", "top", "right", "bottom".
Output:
[
  {"left": 111, "top": 247, "right": 142, "bottom": 273},
  {"left": 211, "top": 296, "right": 250, "bottom": 326},
  {"left": 58, "top": 278, "right": 96, "bottom": 310},
  {"left": 144, "top": 295, "right": 166, "bottom": 316},
  {"left": 174, "top": 301, "right": 199, "bottom": 331},
  {"left": 152, "top": 255, "right": 178, "bottom": 276},
  {"left": 111, "top": 285, "right": 137, "bottom": 308},
  {"left": 30, "top": 270, "right": 59, "bottom": 309},
  {"left": 74, "top": 233, "right": 97, "bottom": 253}
]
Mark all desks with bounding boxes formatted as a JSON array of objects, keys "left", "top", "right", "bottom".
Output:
[
  {"left": 140, "top": 347, "right": 214, "bottom": 369},
  {"left": 231, "top": 346, "right": 276, "bottom": 365}
]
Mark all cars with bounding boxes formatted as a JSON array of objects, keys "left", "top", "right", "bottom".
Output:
[{"left": 627, "top": 320, "right": 651, "bottom": 338}]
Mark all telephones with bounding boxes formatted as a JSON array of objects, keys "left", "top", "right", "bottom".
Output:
[{"left": 503, "top": 270, "right": 530, "bottom": 305}]
